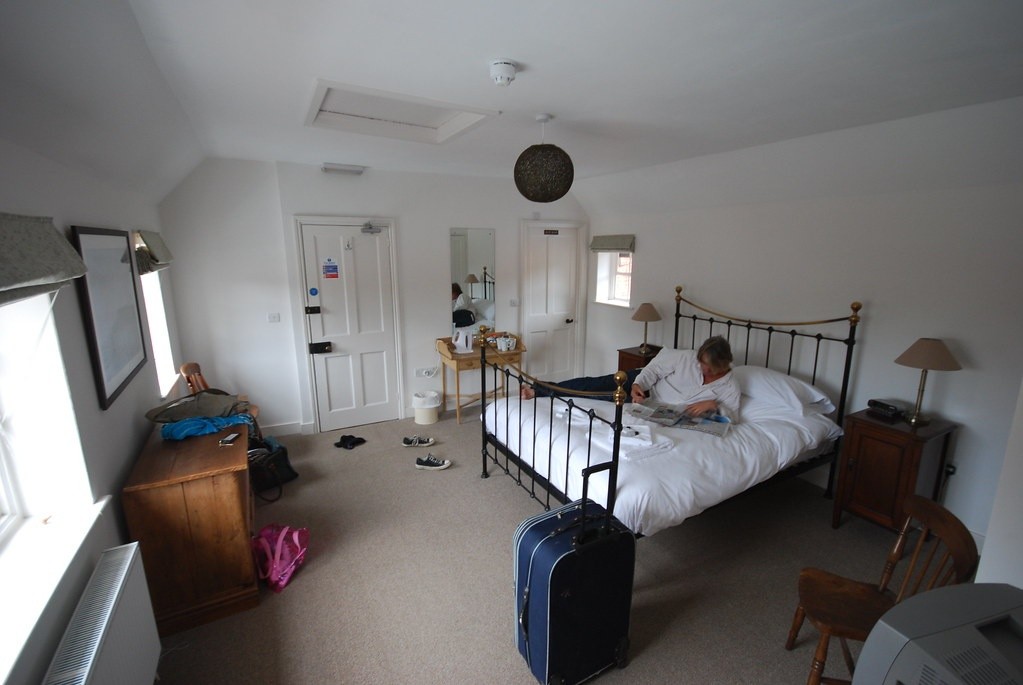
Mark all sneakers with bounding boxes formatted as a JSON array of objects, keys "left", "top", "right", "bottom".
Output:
[
  {"left": 415, "top": 453, "right": 452, "bottom": 470},
  {"left": 402, "top": 434, "right": 435, "bottom": 448}
]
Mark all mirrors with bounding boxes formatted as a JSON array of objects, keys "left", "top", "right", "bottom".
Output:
[{"left": 449, "top": 227, "right": 496, "bottom": 336}]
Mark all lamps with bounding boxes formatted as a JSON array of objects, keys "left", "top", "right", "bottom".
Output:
[
  {"left": 514, "top": 114, "right": 574, "bottom": 203},
  {"left": 464, "top": 274, "right": 480, "bottom": 299},
  {"left": 893, "top": 338, "right": 963, "bottom": 424},
  {"left": 631, "top": 303, "right": 662, "bottom": 353},
  {"left": 320, "top": 162, "right": 364, "bottom": 177}
]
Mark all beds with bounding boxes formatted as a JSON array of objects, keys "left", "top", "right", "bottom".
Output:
[
  {"left": 453, "top": 266, "right": 495, "bottom": 335},
  {"left": 479, "top": 286, "right": 861, "bottom": 537}
]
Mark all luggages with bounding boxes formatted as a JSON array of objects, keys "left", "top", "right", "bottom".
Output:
[{"left": 512, "top": 461, "right": 636, "bottom": 685}]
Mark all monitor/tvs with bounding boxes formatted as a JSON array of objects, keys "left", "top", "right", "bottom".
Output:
[{"left": 851, "top": 582, "right": 1023, "bottom": 685}]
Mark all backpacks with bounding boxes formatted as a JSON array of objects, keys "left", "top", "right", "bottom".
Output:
[{"left": 253, "top": 524, "right": 310, "bottom": 594}]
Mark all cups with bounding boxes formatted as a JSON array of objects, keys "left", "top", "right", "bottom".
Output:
[{"left": 496, "top": 338, "right": 517, "bottom": 351}]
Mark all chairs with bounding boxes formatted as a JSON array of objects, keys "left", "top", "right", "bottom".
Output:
[
  {"left": 786, "top": 494, "right": 979, "bottom": 685},
  {"left": 180, "top": 362, "right": 260, "bottom": 419}
]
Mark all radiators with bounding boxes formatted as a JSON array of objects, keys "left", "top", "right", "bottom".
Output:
[{"left": 41, "top": 541, "right": 162, "bottom": 685}]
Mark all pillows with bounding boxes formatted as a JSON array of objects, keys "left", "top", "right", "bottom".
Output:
[
  {"left": 732, "top": 365, "right": 834, "bottom": 418},
  {"left": 472, "top": 299, "right": 495, "bottom": 321}
]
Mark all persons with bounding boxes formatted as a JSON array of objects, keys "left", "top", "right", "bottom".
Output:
[
  {"left": 452, "top": 282, "right": 476, "bottom": 328},
  {"left": 521, "top": 336, "right": 741, "bottom": 424}
]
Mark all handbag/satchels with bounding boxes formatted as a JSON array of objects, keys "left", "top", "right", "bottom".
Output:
[
  {"left": 241, "top": 435, "right": 299, "bottom": 502},
  {"left": 144, "top": 388, "right": 252, "bottom": 423}
]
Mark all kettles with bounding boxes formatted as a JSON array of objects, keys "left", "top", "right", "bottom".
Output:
[{"left": 451, "top": 330, "right": 473, "bottom": 352}]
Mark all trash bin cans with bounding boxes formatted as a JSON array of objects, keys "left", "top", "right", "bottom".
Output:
[{"left": 412, "top": 392, "right": 440, "bottom": 424}]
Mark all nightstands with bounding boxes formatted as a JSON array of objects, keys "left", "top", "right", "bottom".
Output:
[
  {"left": 832, "top": 407, "right": 960, "bottom": 541},
  {"left": 617, "top": 343, "right": 662, "bottom": 373}
]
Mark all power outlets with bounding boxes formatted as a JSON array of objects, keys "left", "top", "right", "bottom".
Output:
[{"left": 416, "top": 367, "right": 438, "bottom": 377}]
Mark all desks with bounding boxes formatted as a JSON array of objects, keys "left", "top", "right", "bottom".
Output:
[
  {"left": 118, "top": 400, "right": 261, "bottom": 639},
  {"left": 437, "top": 331, "right": 527, "bottom": 424}
]
